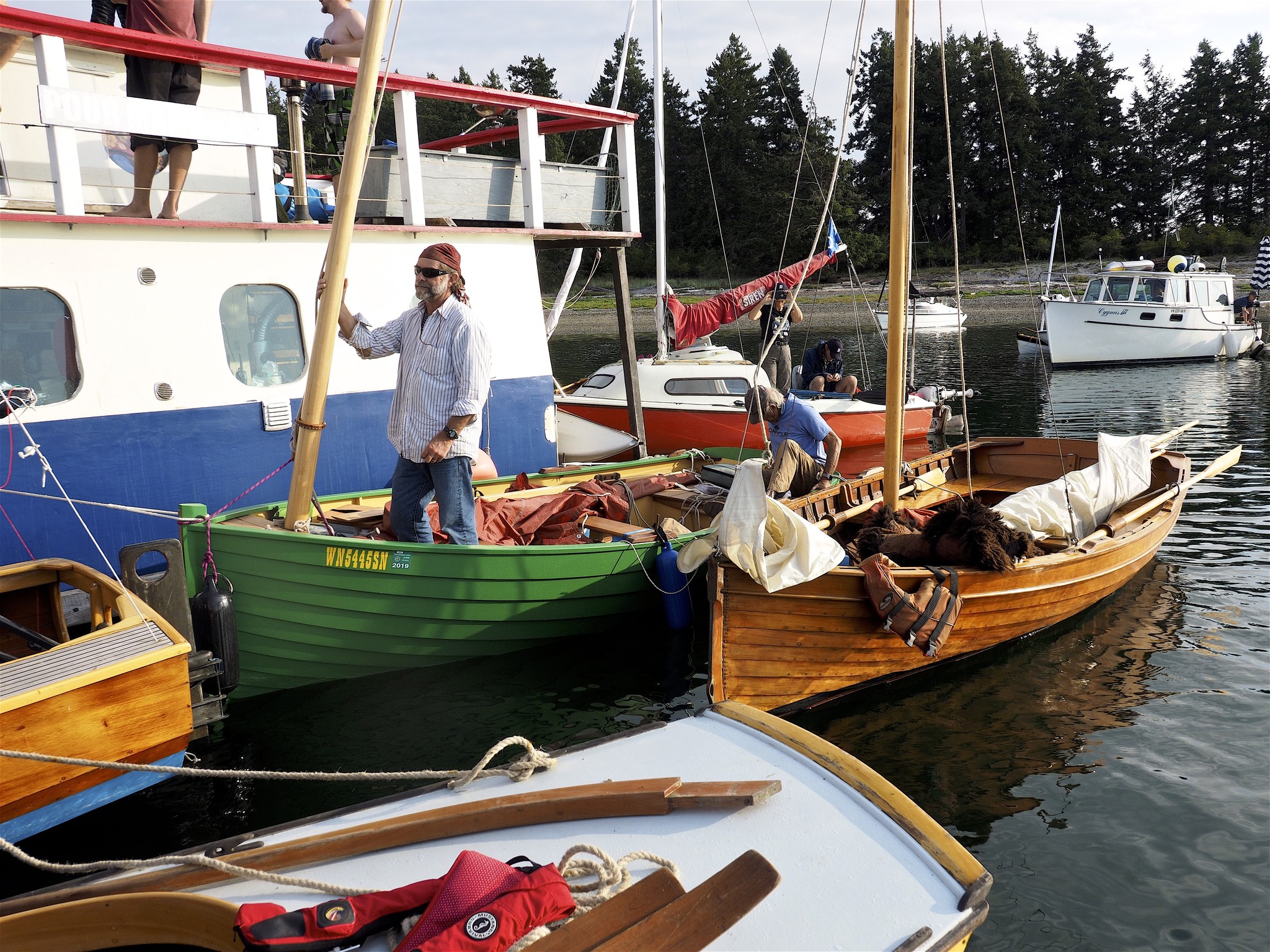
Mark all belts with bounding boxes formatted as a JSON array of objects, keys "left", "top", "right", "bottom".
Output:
[{"left": 760, "top": 339, "right": 789, "bottom": 346}]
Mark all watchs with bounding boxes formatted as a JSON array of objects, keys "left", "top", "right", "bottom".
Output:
[
  {"left": 443, "top": 426, "right": 459, "bottom": 439},
  {"left": 822, "top": 473, "right": 833, "bottom": 481}
]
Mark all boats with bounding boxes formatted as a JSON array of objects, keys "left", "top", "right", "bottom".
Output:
[
  {"left": 1037, "top": 267, "right": 1262, "bottom": 368},
  {"left": 2, "top": 557, "right": 194, "bottom": 848},
  {"left": 0, "top": 697, "right": 989, "bottom": 952},
  {"left": 0, "top": 4, "right": 643, "bottom": 585}
]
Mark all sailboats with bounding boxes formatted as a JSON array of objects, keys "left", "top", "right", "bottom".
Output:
[
  {"left": 554, "top": 1, "right": 946, "bottom": 468},
  {"left": 1015, "top": 206, "right": 1050, "bottom": 354},
  {"left": 873, "top": 217, "right": 973, "bottom": 328},
  {"left": 707, "top": 2, "right": 1192, "bottom": 709},
  {"left": 179, "top": 10, "right": 851, "bottom": 696}
]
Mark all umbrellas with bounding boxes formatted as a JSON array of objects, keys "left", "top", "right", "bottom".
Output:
[{"left": 1249, "top": 235, "right": 1270, "bottom": 290}]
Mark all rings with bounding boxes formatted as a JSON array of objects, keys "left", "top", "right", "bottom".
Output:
[{"left": 431, "top": 459, "right": 437, "bottom": 462}]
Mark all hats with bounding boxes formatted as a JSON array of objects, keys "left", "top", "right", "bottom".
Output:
[
  {"left": 773, "top": 282, "right": 788, "bottom": 292},
  {"left": 744, "top": 385, "right": 768, "bottom": 424},
  {"left": 1249, "top": 291, "right": 1260, "bottom": 297},
  {"left": 419, "top": 243, "right": 462, "bottom": 274},
  {"left": 827, "top": 338, "right": 843, "bottom": 360}
]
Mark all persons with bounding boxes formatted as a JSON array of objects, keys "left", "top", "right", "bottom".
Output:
[
  {"left": 1233, "top": 291, "right": 1260, "bottom": 326},
  {"left": 307, "top": 0, "right": 371, "bottom": 225},
  {"left": 800, "top": 338, "right": 858, "bottom": 398},
  {"left": 110, "top": 0, "right": 212, "bottom": 218},
  {"left": 745, "top": 385, "right": 843, "bottom": 501},
  {"left": 748, "top": 281, "right": 800, "bottom": 392},
  {"left": 316, "top": 245, "right": 496, "bottom": 551}
]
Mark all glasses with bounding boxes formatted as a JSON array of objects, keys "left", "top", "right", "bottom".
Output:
[{"left": 414, "top": 265, "right": 450, "bottom": 278}]
